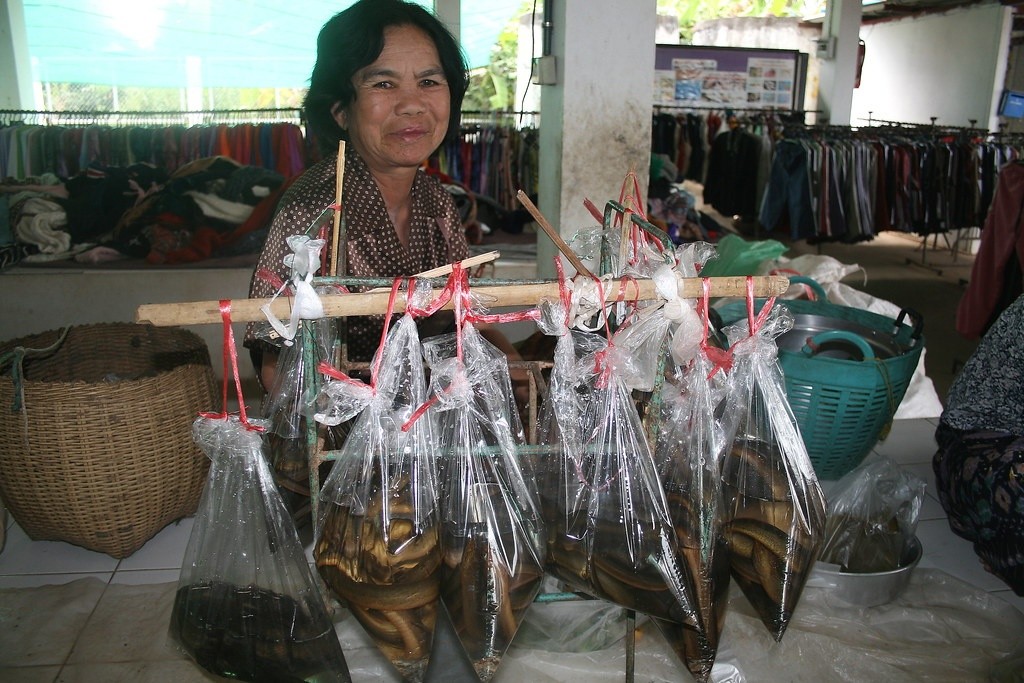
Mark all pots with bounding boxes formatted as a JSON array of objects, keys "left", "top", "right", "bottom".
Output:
[{"left": 708, "top": 308, "right": 924, "bottom": 362}]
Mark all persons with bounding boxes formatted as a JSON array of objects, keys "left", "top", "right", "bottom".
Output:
[
  {"left": 934, "top": 293, "right": 1024, "bottom": 598},
  {"left": 242, "top": 0, "right": 535, "bottom": 449}
]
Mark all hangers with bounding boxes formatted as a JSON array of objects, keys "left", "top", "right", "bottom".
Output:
[{"left": 0, "top": 102, "right": 1024, "bottom": 159}]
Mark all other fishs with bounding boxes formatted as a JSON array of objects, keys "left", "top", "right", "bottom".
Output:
[{"left": 167, "top": 436, "right": 916, "bottom": 683}]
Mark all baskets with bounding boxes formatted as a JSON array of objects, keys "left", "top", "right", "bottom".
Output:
[
  {"left": 716, "top": 273, "right": 924, "bottom": 480},
  {"left": 0, "top": 321, "right": 222, "bottom": 558}
]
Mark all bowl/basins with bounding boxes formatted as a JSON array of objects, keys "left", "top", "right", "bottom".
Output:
[{"left": 809, "top": 535, "right": 923, "bottom": 609}]
